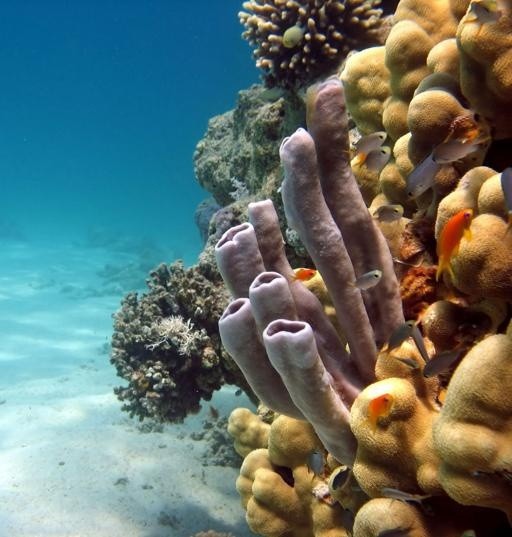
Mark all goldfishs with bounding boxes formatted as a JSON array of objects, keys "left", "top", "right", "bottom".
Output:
[
  {"left": 359, "top": 393, "right": 394, "bottom": 436},
  {"left": 290, "top": 268, "right": 316, "bottom": 284}
]
